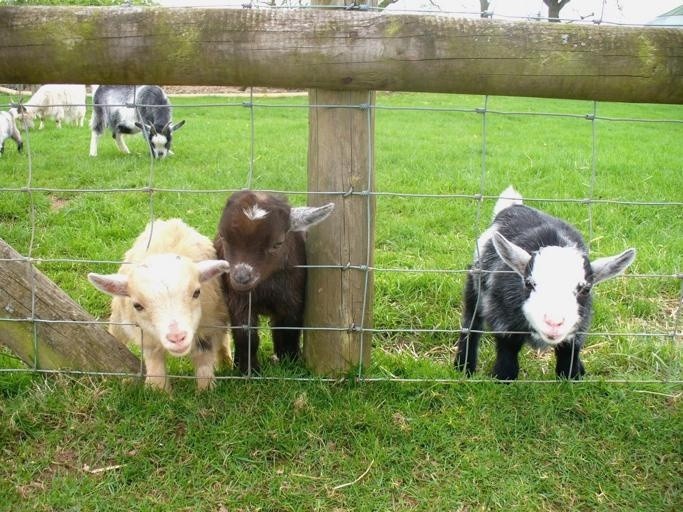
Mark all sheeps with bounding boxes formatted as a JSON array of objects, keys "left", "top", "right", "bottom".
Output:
[
  {"left": 88, "top": 84, "right": 186, "bottom": 162},
  {"left": 18, "top": 84, "right": 86, "bottom": 131},
  {"left": 0, "top": 94, "right": 28, "bottom": 156},
  {"left": 87, "top": 216, "right": 233, "bottom": 399},
  {"left": 214, "top": 189, "right": 335, "bottom": 377},
  {"left": 454, "top": 185, "right": 637, "bottom": 393}
]
